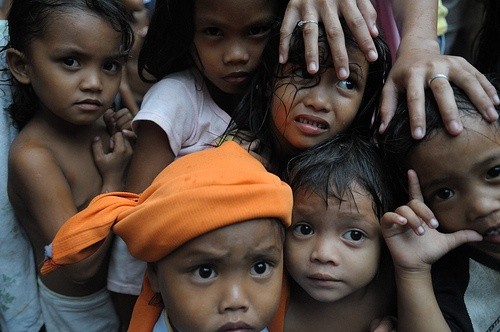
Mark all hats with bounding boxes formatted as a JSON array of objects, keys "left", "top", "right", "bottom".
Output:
[{"left": 41, "top": 140, "right": 297, "bottom": 332}]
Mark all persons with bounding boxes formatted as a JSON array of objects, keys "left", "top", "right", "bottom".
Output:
[
  {"left": 107, "top": 1, "right": 280, "bottom": 332},
  {"left": 280, "top": 1, "right": 499, "bottom": 142},
  {"left": 39, "top": 140, "right": 292, "bottom": 332},
  {"left": 0, "top": 19, "right": 57, "bottom": 332},
  {"left": 0, "top": 0, "right": 145, "bottom": 332},
  {"left": 260, "top": 125, "right": 413, "bottom": 332},
  {"left": 114, "top": 0, "right": 159, "bottom": 119},
  {"left": 212, "top": 14, "right": 391, "bottom": 193},
  {"left": 370, "top": 81, "right": 500, "bottom": 332}
]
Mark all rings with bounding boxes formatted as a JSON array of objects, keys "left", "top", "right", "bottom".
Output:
[
  {"left": 297, "top": 19, "right": 318, "bottom": 30},
  {"left": 427, "top": 74, "right": 449, "bottom": 85}
]
